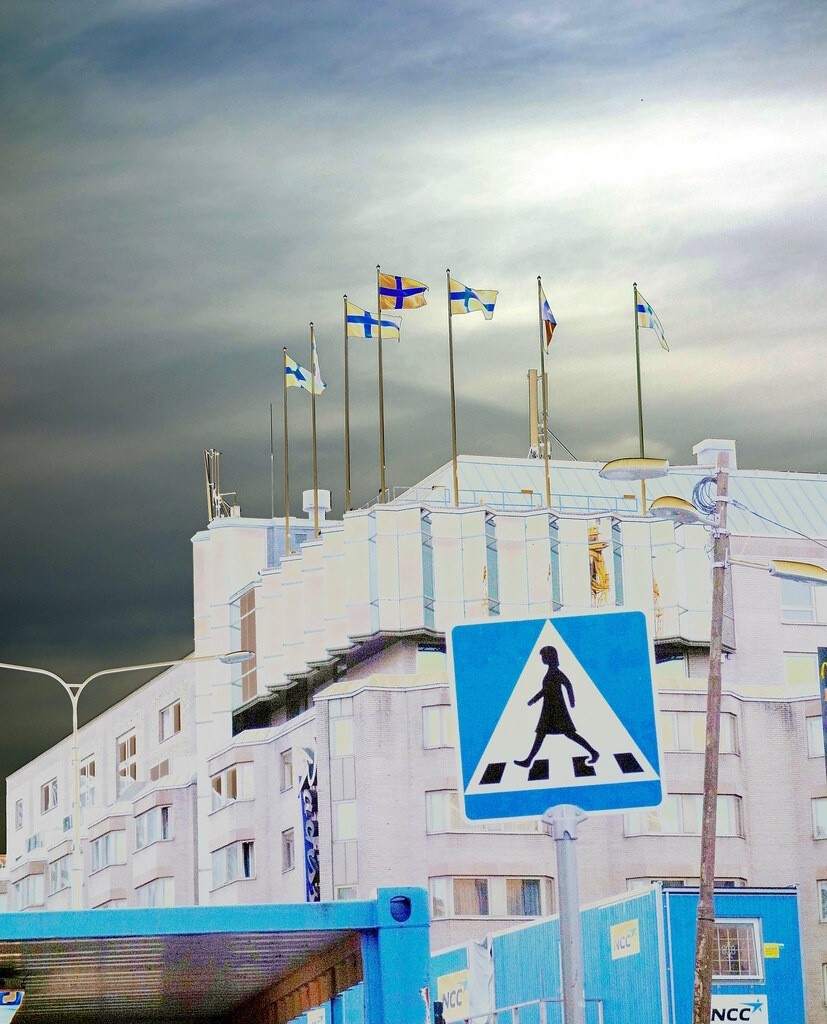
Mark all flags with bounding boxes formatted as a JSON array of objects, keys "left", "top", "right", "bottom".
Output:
[
  {"left": 284, "top": 353, "right": 327, "bottom": 396},
  {"left": 377, "top": 271, "right": 430, "bottom": 310},
  {"left": 540, "top": 282, "right": 558, "bottom": 355},
  {"left": 312, "top": 332, "right": 322, "bottom": 388},
  {"left": 449, "top": 278, "right": 499, "bottom": 321},
  {"left": 635, "top": 290, "right": 670, "bottom": 351},
  {"left": 346, "top": 301, "right": 402, "bottom": 343}
]
